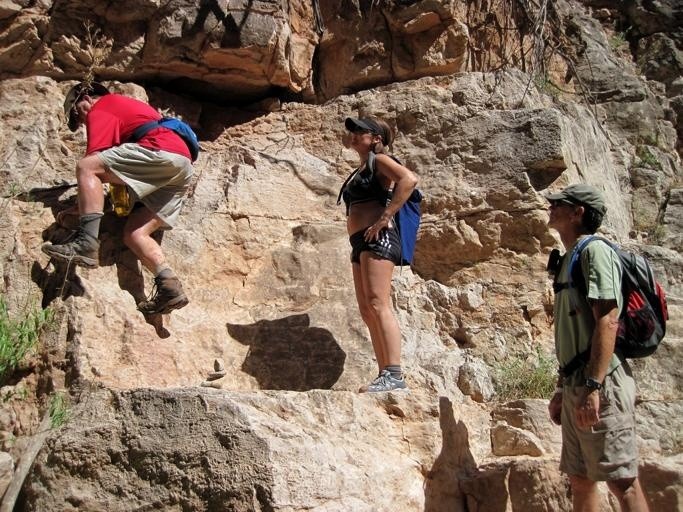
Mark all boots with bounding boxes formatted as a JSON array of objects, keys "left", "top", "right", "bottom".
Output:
[
  {"left": 40, "top": 225, "right": 101, "bottom": 269},
  {"left": 136, "top": 274, "right": 189, "bottom": 314}
]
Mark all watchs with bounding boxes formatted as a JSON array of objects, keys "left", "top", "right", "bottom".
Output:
[{"left": 584, "top": 379, "right": 602, "bottom": 390}]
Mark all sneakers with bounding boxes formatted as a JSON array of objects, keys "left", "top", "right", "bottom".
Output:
[{"left": 358, "top": 369, "right": 410, "bottom": 394}]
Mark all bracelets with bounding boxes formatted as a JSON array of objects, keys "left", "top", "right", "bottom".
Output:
[{"left": 554, "top": 387, "right": 564, "bottom": 394}]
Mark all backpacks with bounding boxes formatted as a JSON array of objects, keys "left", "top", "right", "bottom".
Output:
[
  {"left": 569, "top": 235, "right": 670, "bottom": 359},
  {"left": 366, "top": 151, "right": 423, "bottom": 267}
]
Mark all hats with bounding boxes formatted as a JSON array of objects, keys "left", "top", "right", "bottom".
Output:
[
  {"left": 544, "top": 183, "right": 608, "bottom": 216},
  {"left": 62, "top": 81, "right": 110, "bottom": 133},
  {"left": 344, "top": 116, "right": 388, "bottom": 147}
]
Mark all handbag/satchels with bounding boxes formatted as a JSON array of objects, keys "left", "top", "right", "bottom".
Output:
[{"left": 160, "top": 116, "right": 198, "bottom": 161}]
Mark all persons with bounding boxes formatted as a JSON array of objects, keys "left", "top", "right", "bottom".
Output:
[
  {"left": 341, "top": 117, "right": 418, "bottom": 392},
  {"left": 543, "top": 182, "right": 649, "bottom": 512},
  {"left": 41, "top": 80, "right": 193, "bottom": 316}
]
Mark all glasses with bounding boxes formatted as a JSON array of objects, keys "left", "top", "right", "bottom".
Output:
[{"left": 549, "top": 199, "right": 575, "bottom": 207}]
